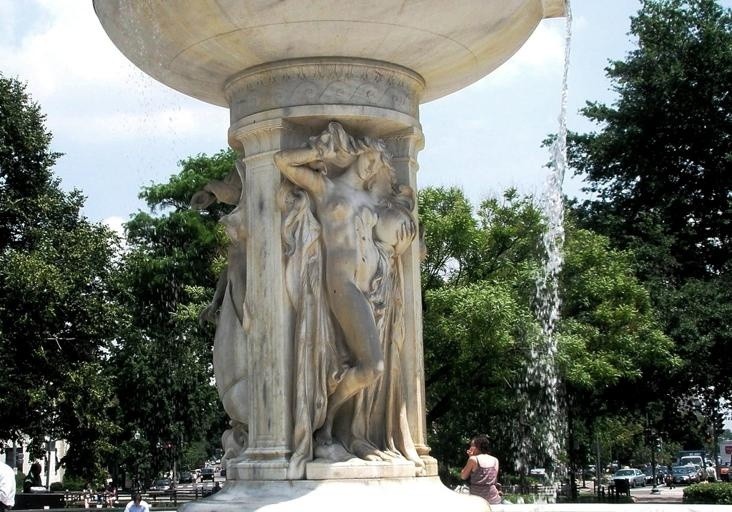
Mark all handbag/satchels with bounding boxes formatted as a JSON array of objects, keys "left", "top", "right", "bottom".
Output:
[{"left": 453, "top": 485, "right": 471, "bottom": 495}]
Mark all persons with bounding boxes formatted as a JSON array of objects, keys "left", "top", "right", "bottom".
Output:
[
  {"left": 607, "top": 478, "right": 616, "bottom": 495},
  {"left": 211, "top": 481, "right": 222, "bottom": 496},
  {"left": 706, "top": 462, "right": 718, "bottom": 483},
  {"left": 0, "top": 459, "right": 17, "bottom": 512},
  {"left": 270, "top": 118, "right": 416, "bottom": 465},
  {"left": 459, "top": 432, "right": 503, "bottom": 506},
  {"left": 80, "top": 483, "right": 92, "bottom": 510},
  {"left": 187, "top": 152, "right": 250, "bottom": 463},
  {"left": 105, "top": 482, "right": 118, "bottom": 509},
  {"left": 123, "top": 493, "right": 149, "bottom": 512}
]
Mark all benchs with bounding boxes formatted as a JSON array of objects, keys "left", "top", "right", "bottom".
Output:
[{"left": 65, "top": 487, "right": 214, "bottom": 507}]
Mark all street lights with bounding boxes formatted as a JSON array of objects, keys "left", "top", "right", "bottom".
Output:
[
  {"left": 131, "top": 429, "right": 141, "bottom": 488},
  {"left": 651, "top": 425, "right": 659, "bottom": 492}
]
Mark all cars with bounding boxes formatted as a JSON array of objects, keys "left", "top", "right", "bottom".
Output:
[
  {"left": 577, "top": 439, "right": 732, "bottom": 488},
  {"left": 147, "top": 451, "right": 227, "bottom": 501}
]
